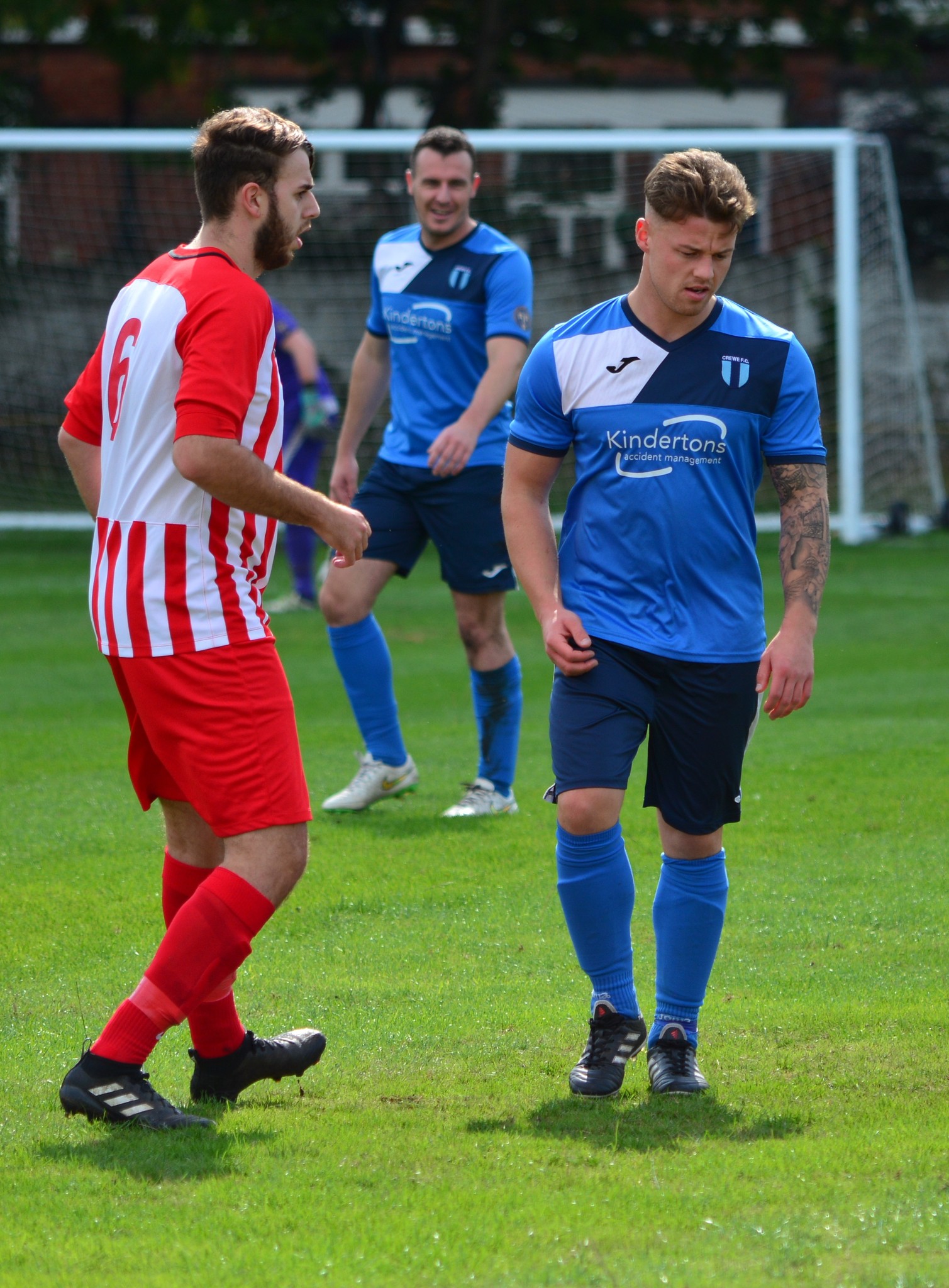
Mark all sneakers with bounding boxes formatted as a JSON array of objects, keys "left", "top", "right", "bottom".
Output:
[
  {"left": 439, "top": 777, "right": 518, "bottom": 817},
  {"left": 191, "top": 1028, "right": 327, "bottom": 1102},
  {"left": 60, "top": 1052, "right": 217, "bottom": 1130},
  {"left": 322, "top": 753, "right": 420, "bottom": 813},
  {"left": 570, "top": 1000, "right": 646, "bottom": 1096},
  {"left": 646, "top": 1023, "right": 709, "bottom": 1094},
  {"left": 260, "top": 591, "right": 317, "bottom": 613}
]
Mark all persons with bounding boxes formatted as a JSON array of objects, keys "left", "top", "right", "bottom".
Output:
[
  {"left": 494, "top": 144, "right": 831, "bottom": 1100},
  {"left": 250, "top": 274, "right": 341, "bottom": 615},
  {"left": 321, "top": 125, "right": 533, "bottom": 818},
  {"left": 57, "top": 103, "right": 371, "bottom": 1137}
]
